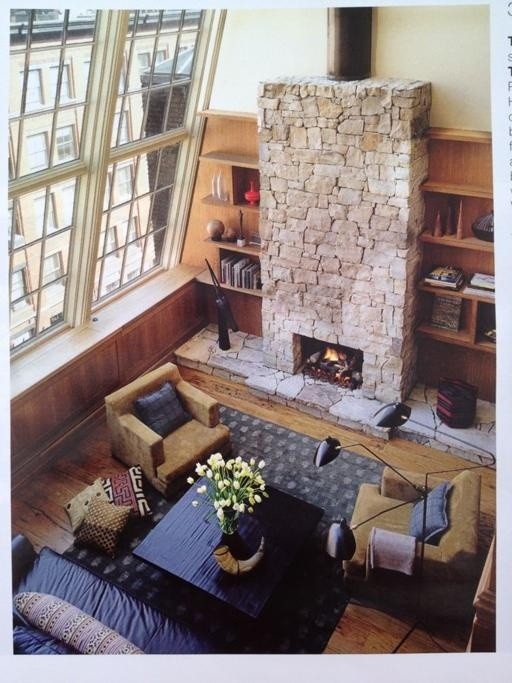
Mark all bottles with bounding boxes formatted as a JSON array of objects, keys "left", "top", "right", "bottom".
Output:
[
  {"left": 211, "top": 166, "right": 228, "bottom": 202},
  {"left": 242, "top": 178, "right": 260, "bottom": 207}
]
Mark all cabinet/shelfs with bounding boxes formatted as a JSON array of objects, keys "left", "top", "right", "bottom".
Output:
[
  {"left": 416, "top": 132, "right": 496, "bottom": 356},
  {"left": 195, "top": 112, "right": 262, "bottom": 297}
]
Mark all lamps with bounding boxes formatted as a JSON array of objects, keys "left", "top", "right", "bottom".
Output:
[{"left": 315, "top": 403, "right": 496, "bottom": 654}]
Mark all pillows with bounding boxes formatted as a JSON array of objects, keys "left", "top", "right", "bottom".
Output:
[
  {"left": 64, "top": 464, "right": 155, "bottom": 559},
  {"left": 133, "top": 382, "right": 194, "bottom": 439},
  {"left": 410, "top": 481, "right": 454, "bottom": 543},
  {"left": 14, "top": 591, "right": 146, "bottom": 654}
]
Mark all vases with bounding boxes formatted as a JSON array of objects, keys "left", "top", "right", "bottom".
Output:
[{"left": 220, "top": 510, "right": 241, "bottom": 535}]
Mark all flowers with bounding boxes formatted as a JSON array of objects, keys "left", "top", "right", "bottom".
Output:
[{"left": 187, "top": 453, "right": 271, "bottom": 535}]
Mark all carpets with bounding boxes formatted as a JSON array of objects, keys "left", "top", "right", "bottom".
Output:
[{"left": 63, "top": 402, "right": 388, "bottom": 654}]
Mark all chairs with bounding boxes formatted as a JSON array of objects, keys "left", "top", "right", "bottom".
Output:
[
  {"left": 104, "top": 362, "right": 233, "bottom": 501},
  {"left": 347, "top": 466, "right": 477, "bottom": 620}
]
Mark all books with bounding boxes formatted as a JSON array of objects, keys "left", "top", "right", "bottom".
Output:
[
  {"left": 425, "top": 265, "right": 494, "bottom": 348},
  {"left": 220, "top": 257, "right": 261, "bottom": 291}
]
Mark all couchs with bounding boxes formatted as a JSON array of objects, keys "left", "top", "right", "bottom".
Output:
[{"left": 12, "top": 533, "right": 217, "bottom": 654}]
